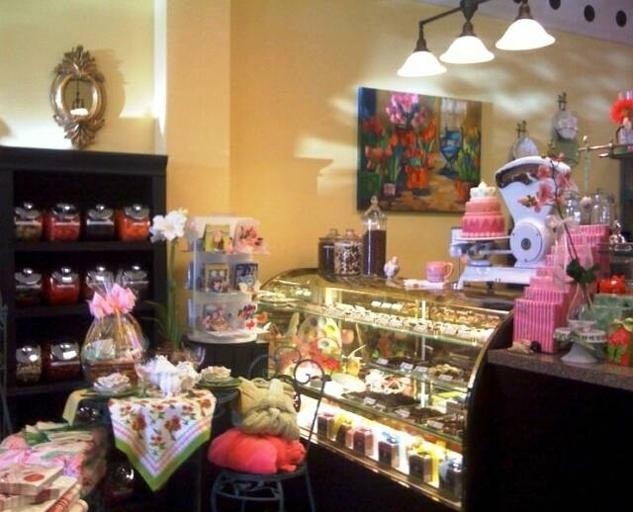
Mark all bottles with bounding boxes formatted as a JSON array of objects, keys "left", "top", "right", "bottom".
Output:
[
  {"left": 362, "top": 194, "right": 388, "bottom": 281},
  {"left": 47, "top": 338, "right": 82, "bottom": 383},
  {"left": 13, "top": 266, "right": 152, "bottom": 304},
  {"left": 334, "top": 229, "right": 363, "bottom": 274},
  {"left": 13, "top": 343, "right": 44, "bottom": 385},
  {"left": 559, "top": 192, "right": 618, "bottom": 227},
  {"left": 315, "top": 229, "right": 342, "bottom": 279},
  {"left": 13, "top": 198, "right": 152, "bottom": 244}
]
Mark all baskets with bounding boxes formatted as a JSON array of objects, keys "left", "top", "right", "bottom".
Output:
[{"left": 79, "top": 312, "right": 145, "bottom": 388}]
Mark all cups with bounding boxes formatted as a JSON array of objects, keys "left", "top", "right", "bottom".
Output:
[{"left": 425, "top": 259, "right": 454, "bottom": 283}]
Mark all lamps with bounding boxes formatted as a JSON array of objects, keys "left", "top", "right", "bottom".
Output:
[
  {"left": 397, "top": 1, "right": 562, "bottom": 80},
  {"left": 548, "top": 91, "right": 580, "bottom": 169},
  {"left": 510, "top": 120, "right": 540, "bottom": 156},
  {"left": 67, "top": 80, "right": 87, "bottom": 114}
]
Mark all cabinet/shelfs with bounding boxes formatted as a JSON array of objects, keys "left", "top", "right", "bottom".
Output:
[
  {"left": 0, "top": 145, "right": 170, "bottom": 440},
  {"left": 247, "top": 267, "right": 520, "bottom": 512}
]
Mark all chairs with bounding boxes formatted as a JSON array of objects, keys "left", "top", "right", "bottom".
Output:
[{"left": 206, "top": 345, "right": 326, "bottom": 508}]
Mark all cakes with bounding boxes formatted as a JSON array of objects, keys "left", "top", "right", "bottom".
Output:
[{"left": 461, "top": 180, "right": 506, "bottom": 237}]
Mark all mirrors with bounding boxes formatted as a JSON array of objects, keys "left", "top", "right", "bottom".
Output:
[{"left": 49, "top": 45, "right": 107, "bottom": 148}]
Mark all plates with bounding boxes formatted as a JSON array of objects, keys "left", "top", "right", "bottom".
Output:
[
  {"left": 554, "top": 109, "right": 579, "bottom": 140},
  {"left": 85, "top": 386, "right": 140, "bottom": 397},
  {"left": 512, "top": 138, "right": 538, "bottom": 162},
  {"left": 193, "top": 376, "right": 243, "bottom": 389}
]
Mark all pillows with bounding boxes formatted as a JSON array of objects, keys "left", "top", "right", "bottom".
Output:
[{"left": 236, "top": 377, "right": 298, "bottom": 438}]
